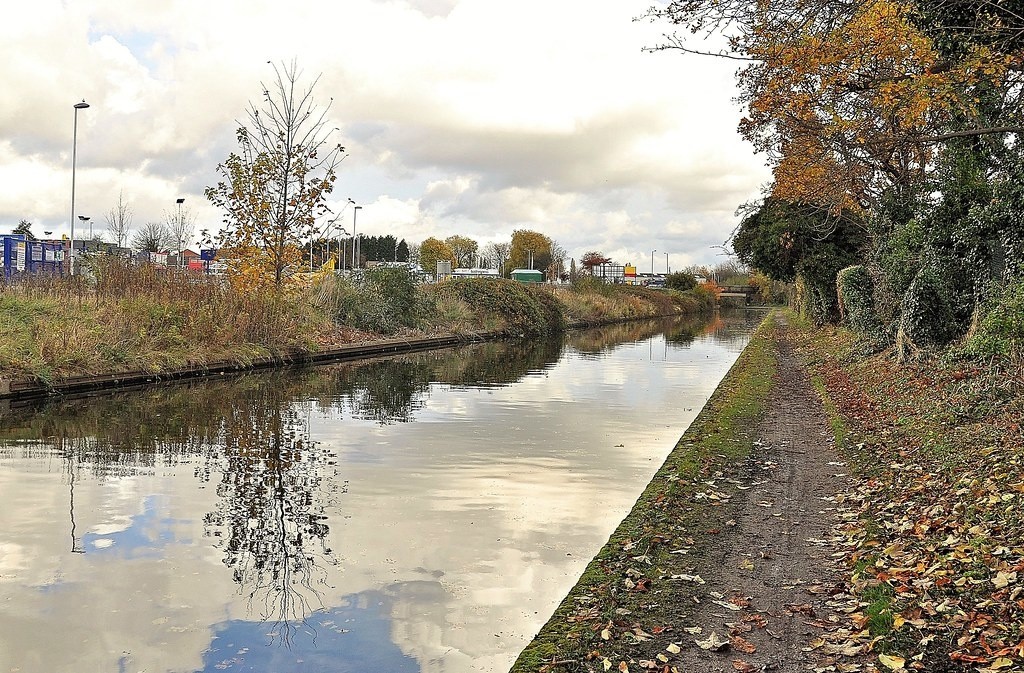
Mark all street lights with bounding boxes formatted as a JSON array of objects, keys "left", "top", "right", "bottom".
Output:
[
  {"left": 70, "top": 100, "right": 90, "bottom": 274},
  {"left": 326, "top": 220, "right": 333, "bottom": 261},
  {"left": 176, "top": 198, "right": 186, "bottom": 270},
  {"left": 335, "top": 227, "right": 340, "bottom": 271},
  {"left": 664, "top": 252, "right": 668, "bottom": 276},
  {"left": 651, "top": 250, "right": 657, "bottom": 274},
  {"left": 339, "top": 228, "right": 345, "bottom": 271},
  {"left": 351, "top": 206, "right": 363, "bottom": 268}
]
